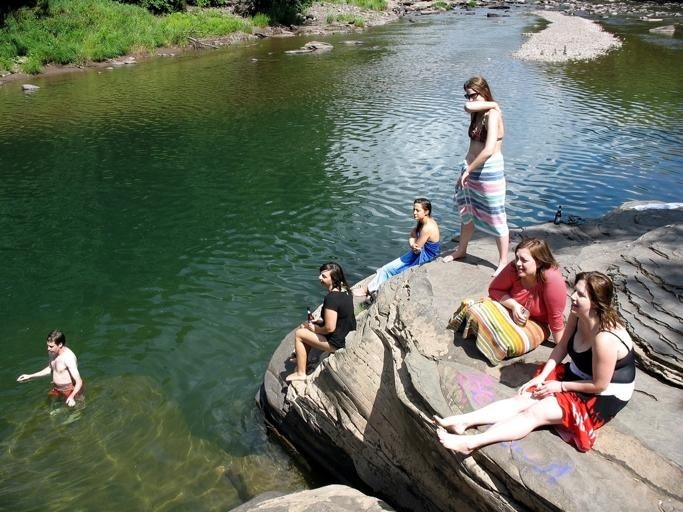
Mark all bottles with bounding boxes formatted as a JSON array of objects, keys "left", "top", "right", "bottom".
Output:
[
  {"left": 554, "top": 206, "right": 562, "bottom": 225},
  {"left": 307, "top": 307, "right": 315, "bottom": 326}
]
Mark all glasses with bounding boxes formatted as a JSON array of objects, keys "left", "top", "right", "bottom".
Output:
[{"left": 464, "top": 93, "right": 479, "bottom": 99}]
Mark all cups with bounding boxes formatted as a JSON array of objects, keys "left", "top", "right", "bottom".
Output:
[{"left": 520, "top": 306, "right": 530, "bottom": 326}]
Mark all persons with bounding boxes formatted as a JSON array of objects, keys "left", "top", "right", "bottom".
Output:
[
  {"left": 283, "top": 261, "right": 357, "bottom": 381},
  {"left": 14, "top": 329, "right": 83, "bottom": 410},
  {"left": 442, "top": 75, "right": 510, "bottom": 281},
  {"left": 433, "top": 271, "right": 636, "bottom": 457},
  {"left": 446, "top": 237, "right": 568, "bottom": 369},
  {"left": 351, "top": 197, "right": 442, "bottom": 297}
]
